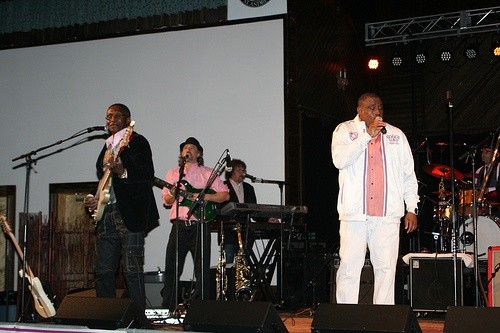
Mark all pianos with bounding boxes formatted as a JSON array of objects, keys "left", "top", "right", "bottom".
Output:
[{"left": 221, "top": 202, "right": 308, "bottom": 304}]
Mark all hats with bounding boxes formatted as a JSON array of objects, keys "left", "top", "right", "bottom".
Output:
[{"left": 180, "top": 137, "right": 203, "bottom": 155}]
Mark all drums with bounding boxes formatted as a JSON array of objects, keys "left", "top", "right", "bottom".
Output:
[
  {"left": 457, "top": 187, "right": 486, "bottom": 215},
  {"left": 457, "top": 213, "right": 500, "bottom": 259},
  {"left": 433, "top": 202, "right": 453, "bottom": 221}
]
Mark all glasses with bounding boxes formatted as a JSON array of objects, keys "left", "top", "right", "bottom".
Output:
[{"left": 105, "top": 113, "right": 130, "bottom": 121}]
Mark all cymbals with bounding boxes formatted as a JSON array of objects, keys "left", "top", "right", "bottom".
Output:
[{"left": 424, "top": 163, "right": 464, "bottom": 180}]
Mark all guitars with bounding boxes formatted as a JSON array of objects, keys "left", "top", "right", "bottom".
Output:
[
  {"left": 154, "top": 176, "right": 222, "bottom": 223},
  {"left": 92, "top": 120, "right": 135, "bottom": 221},
  {"left": 0, "top": 216, "right": 56, "bottom": 319}
]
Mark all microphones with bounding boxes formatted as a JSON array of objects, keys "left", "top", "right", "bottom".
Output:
[
  {"left": 241, "top": 172, "right": 256, "bottom": 180},
  {"left": 225, "top": 149, "right": 233, "bottom": 172},
  {"left": 88, "top": 126, "right": 108, "bottom": 131},
  {"left": 376, "top": 116, "right": 387, "bottom": 134},
  {"left": 185, "top": 153, "right": 190, "bottom": 158}
]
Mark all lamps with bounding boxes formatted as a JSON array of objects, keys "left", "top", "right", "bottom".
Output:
[
  {"left": 415, "top": 41, "right": 427, "bottom": 65},
  {"left": 439, "top": 39, "right": 455, "bottom": 64},
  {"left": 463, "top": 35, "right": 485, "bottom": 60},
  {"left": 391, "top": 45, "right": 408, "bottom": 70},
  {"left": 490, "top": 33, "right": 500, "bottom": 57}
]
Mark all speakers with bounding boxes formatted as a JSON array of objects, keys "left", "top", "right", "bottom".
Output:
[
  {"left": 310, "top": 245, "right": 500, "bottom": 333},
  {"left": 183, "top": 299, "right": 290, "bottom": 333},
  {"left": 53, "top": 295, "right": 154, "bottom": 331}
]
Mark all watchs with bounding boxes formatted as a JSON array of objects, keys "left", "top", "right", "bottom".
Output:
[{"left": 408, "top": 207, "right": 418, "bottom": 215}]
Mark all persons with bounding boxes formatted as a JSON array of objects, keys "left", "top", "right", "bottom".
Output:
[
  {"left": 219, "top": 159, "right": 279, "bottom": 303},
  {"left": 160, "top": 137, "right": 230, "bottom": 311},
  {"left": 331, "top": 92, "right": 421, "bottom": 304},
  {"left": 83, "top": 103, "right": 160, "bottom": 330},
  {"left": 474, "top": 142, "right": 500, "bottom": 218}
]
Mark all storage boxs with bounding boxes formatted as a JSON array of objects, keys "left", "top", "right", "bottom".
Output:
[{"left": 410, "top": 253, "right": 463, "bottom": 318}]
